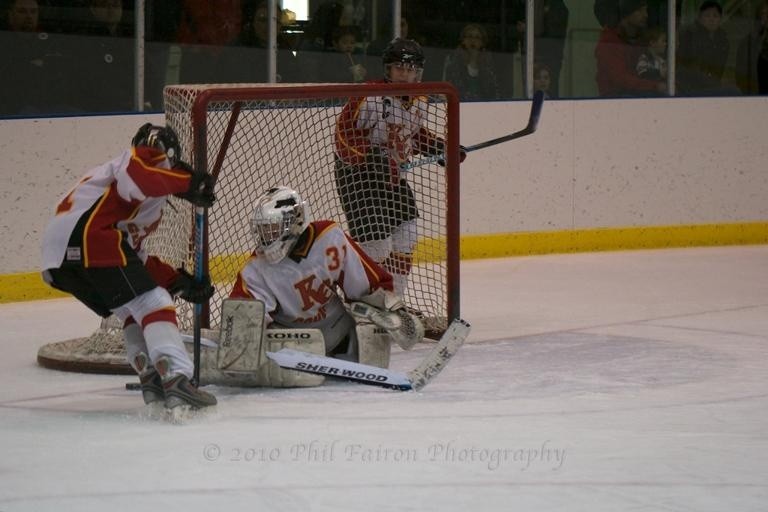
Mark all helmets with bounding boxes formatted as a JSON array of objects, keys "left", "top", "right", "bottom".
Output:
[
  {"left": 131, "top": 122, "right": 180, "bottom": 168},
  {"left": 249, "top": 185, "right": 310, "bottom": 266},
  {"left": 381, "top": 37, "right": 425, "bottom": 83}
]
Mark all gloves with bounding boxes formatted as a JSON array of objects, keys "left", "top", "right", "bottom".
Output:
[
  {"left": 363, "top": 148, "right": 398, "bottom": 187},
  {"left": 425, "top": 137, "right": 466, "bottom": 166},
  {"left": 171, "top": 160, "right": 215, "bottom": 207},
  {"left": 167, "top": 267, "right": 215, "bottom": 303}
]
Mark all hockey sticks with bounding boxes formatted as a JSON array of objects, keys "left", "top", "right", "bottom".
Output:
[
  {"left": 402, "top": 90, "right": 545, "bottom": 170},
  {"left": 180, "top": 316, "right": 471, "bottom": 393}
]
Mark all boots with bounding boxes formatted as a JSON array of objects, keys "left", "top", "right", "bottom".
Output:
[{"left": 131, "top": 351, "right": 217, "bottom": 410}]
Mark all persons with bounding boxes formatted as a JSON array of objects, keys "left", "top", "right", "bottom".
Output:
[
  {"left": 219, "top": 186, "right": 424, "bottom": 390},
  {"left": 335, "top": 35, "right": 467, "bottom": 304},
  {"left": 42, "top": 122, "right": 218, "bottom": 408}
]
